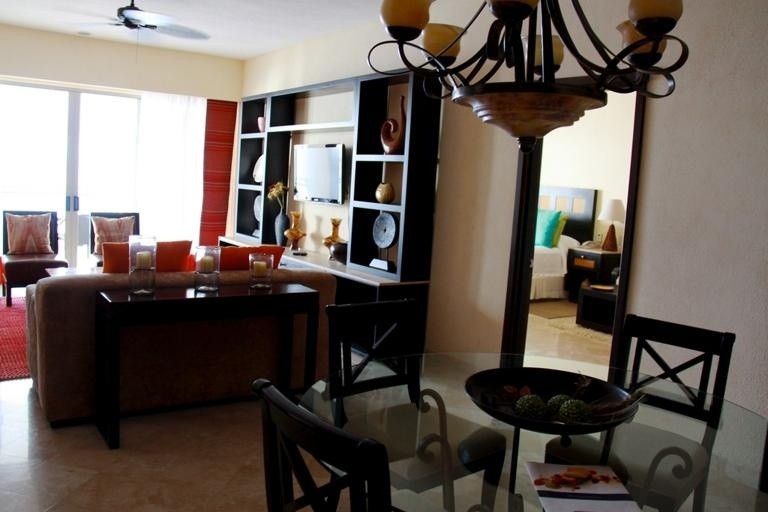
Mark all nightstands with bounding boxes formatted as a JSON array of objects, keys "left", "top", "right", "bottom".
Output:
[{"left": 565, "top": 245, "right": 620, "bottom": 304}]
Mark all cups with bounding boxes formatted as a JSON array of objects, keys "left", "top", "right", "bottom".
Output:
[
  {"left": 193, "top": 245, "right": 221, "bottom": 292},
  {"left": 248, "top": 252, "right": 273, "bottom": 289},
  {"left": 127, "top": 234, "right": 158, "bottom": 296}
]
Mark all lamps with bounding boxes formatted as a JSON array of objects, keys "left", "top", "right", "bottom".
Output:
[
  {"left": 599, "top": 199, "right": 625, "bottom": 251},
  {"left": 367, "top": 0, "right": 690, "bottom": 156}
]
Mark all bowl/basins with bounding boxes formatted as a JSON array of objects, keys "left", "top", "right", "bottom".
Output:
[
  {"left": 257, "top": 116, "right": 265, "bottom": 132},
  {"left": 455, "top": 418, "right": 628, "bottom": 494},
  {"left": 462, "top": 367, "right": 638, "bottom": 436}
]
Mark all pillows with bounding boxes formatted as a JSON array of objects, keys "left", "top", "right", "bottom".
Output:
[
  {"left": 90, "top": 215, "right": 135, "bottom": 257},
  {"left": 534, "top": 208, "right": 568, "bottom": 248},
  {"left": 100, "top": 240, "right": 286, "bottom": 276},
  {"left": 4, "top": 212, "right": 55, "bottom": 255}
]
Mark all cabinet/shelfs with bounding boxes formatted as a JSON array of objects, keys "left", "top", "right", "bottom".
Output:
[
  {"left": 218, "top": 65, "right": 442, "bottom": 363},
  {"left": 577, "top": 284, "right": 618, "bottom": 335}
]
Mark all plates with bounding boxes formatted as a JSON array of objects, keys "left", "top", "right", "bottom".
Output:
[
  {"left": 252, "top": 153, "right": 265, "bottom": 183},
  {"left": 590, "top": 285, "right": 615, "bottom": 290},
  {"left": 372, "top": 212, "right": 396, "bottom": 249}
]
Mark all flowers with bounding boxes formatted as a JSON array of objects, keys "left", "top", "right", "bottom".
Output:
[{"left": 267, "top": 181, "right": 290, "bottom": 208}]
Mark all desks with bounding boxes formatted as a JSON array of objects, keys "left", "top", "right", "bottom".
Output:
[
  {"left": 90, "top": 282, "right": 320, "bottom": 449},
  {"left": 300, "top": 353, "right": 768, "bottom": 512}
]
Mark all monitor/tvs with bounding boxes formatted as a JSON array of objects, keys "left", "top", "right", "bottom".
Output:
[{"left": 293, "top": 142, "right": 347, "bottom": 207}]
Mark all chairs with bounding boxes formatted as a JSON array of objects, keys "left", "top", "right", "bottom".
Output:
[
  {"left": 0, "top": 210, "right": 69, "bottom": 307},
  {"left": 88, "top": 212, "right": 140, "bottom": 267}
]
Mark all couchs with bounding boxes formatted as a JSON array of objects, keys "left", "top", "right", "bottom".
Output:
[{"left": 23, "top": 269, "right": 337, "bottom": 431}]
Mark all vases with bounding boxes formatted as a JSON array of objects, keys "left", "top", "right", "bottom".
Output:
[
  {"left": 285, "top": 211, "right": 306, "bottom": 251},
  {"left": 273, "top": 207, "right": 291, "bottom": 248},
  {"left": 321, "top": 217, "right": 346, "bottom": 259}
]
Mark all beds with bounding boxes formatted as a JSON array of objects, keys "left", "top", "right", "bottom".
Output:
[{"left": 530, "top": 184, "right": 597, "bottom": 300}]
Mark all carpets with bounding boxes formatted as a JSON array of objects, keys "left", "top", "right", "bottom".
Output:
[{"left": 528, "top": 298, "right": 578, "bottom": 319}]
